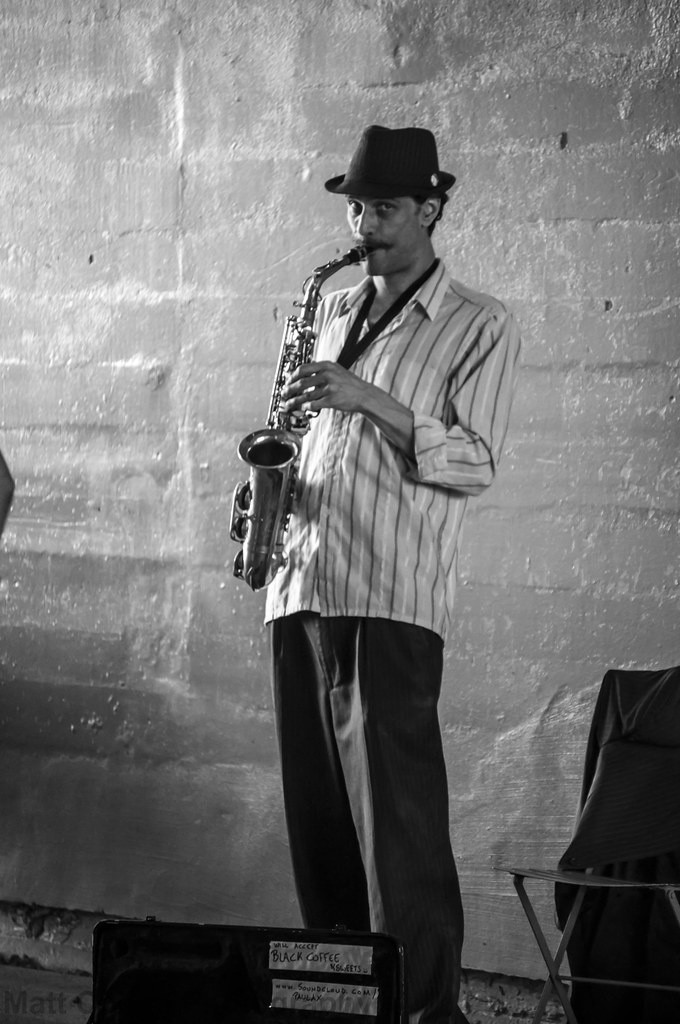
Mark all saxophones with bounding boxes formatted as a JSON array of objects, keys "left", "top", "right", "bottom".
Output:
[{"left": 230, "top": 244, "right": 372, "bottom": 592}]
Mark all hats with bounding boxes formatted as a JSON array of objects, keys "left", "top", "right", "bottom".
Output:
[{"left": 324, "top": 124, "right": 456, "bottom": 198}]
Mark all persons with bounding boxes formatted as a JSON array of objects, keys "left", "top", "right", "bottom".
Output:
[{"left": 263, "top": 124, "right": 518, "bottom": 1024}]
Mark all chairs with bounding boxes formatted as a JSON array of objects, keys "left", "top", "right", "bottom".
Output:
[{"left": 490, "top": 666, "right": 680, "bottom": 1024}]
